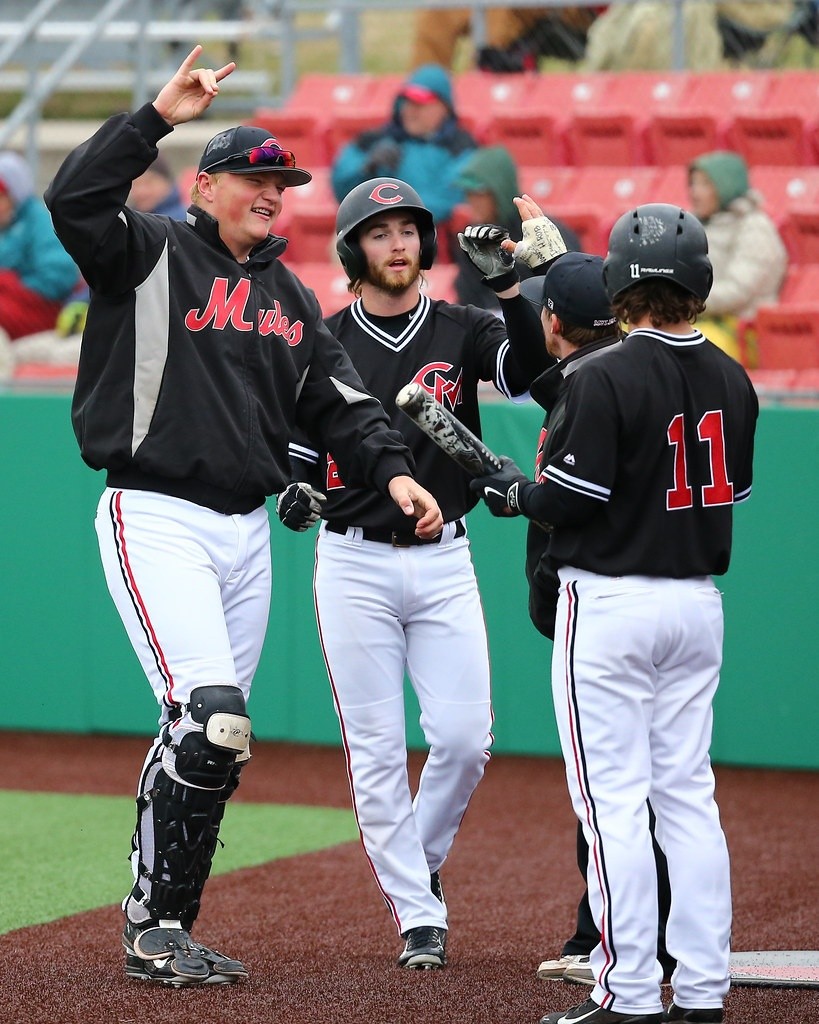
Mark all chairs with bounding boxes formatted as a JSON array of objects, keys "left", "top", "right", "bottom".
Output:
[{"left": 11, "top": 71, "right": 819, "bottom": 407}]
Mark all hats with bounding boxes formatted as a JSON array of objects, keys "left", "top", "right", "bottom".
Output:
[
  {"left": 398, "top": 84, "right": 437, "bottom": 106},
  {"left": 196, "top": 126, "right": 312, "bottom": 186},
  {"left": 518, "top": 251, "right": 620, "bottom": 329}
]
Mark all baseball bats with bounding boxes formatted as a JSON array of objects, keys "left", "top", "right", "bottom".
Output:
[{"left": 395, "top": 382, "right": 553, "bottom": 534}]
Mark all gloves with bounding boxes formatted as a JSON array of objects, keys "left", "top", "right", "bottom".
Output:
[
  {"left": 275, "top": 482, "right": 327, "bottom": 533},
  {"left": 469, "top": 454, "right": 536, "bottom": 517},
  {"left": 457, "top": 224, "right": 519, "bottom": 293},
  {"left": 55, "top": 300, "right": 89, "bottom": 337}
]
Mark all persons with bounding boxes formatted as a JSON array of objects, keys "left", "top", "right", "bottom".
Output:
[
  {"left": 499, "top": 192, "right": 677, "bottom": 986},
  {"left": 43, "top": 44, "right": 446, "bottom": 986},
  {"left": 274, "top": 174, "right": 541, "bottom": 969},
  {"left": 0, "top": 0, "right": 819, "bottom": 365},
  {"left": 472, "top": 201, "right": 761, "bottom": 1024}
]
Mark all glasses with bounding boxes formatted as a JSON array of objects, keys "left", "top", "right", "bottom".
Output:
[{"left": 203, "top": 147, "right": 295, "bottom": 168}]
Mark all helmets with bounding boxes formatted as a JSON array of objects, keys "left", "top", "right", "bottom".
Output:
[
  {"left": 335, "top": 177, "right": 437, "bottom": 280},
  {"left": 602, "top": 203, "right": 713, "bottom": 303}
]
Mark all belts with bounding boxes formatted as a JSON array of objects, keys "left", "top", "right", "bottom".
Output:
[{"left": 325, "top": 519, "right": 465, "bottom": 548}]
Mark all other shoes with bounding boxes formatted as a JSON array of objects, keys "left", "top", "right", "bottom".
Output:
[
  {"left": 477, "top": 38, "right": 536, "bottom": 74},
  {"left": 532, "top": 17, "right": 585, "bottom": 64}
]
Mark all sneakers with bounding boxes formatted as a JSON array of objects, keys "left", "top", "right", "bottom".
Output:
[
  {"left": 124, "top": 923, "right": 239, "bottom": 989},
  {"left": 537, "top": 955, "right": 590, "bottom": 981},
  {"left": 662, "top": 1002, "right": 723, "bottom": 1024},
  {"left": 540, "top": 996, "right": 664, "bottom": 1024},
  {"left": 430, "top": 869, "right": 445, "bottom": 905},
  {"left": 398, "top": 926, "right": 446, "bottom": 970},
  {"left": 563, "top": 956, "right": 672, "bottom": 986}
]
